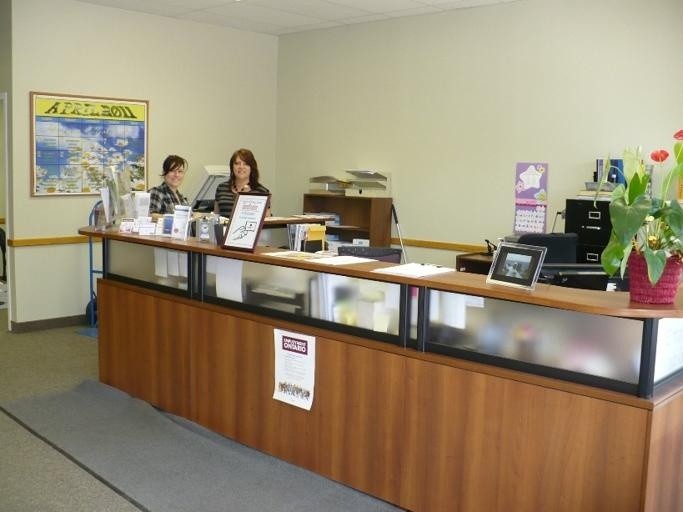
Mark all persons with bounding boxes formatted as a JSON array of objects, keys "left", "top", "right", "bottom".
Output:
[
  {"left": 148, "top": 155, "right": 202, "bottom": 221},
  {"left": 505, "top": 261, "right": 521, "bottom": 278},
  {"left": 214, "top": 151, "right": 273, "bottom": 218}
]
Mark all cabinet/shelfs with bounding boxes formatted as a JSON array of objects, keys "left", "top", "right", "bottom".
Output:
[{"left": 304, "top": 194, "right": 393, "bottom": 249}]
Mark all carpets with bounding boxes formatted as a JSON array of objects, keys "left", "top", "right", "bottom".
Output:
[{"left": 0, "top": 379, "right": 409, "bottom": 511}]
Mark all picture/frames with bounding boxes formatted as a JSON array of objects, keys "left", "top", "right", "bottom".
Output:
[
  {"left": 486, "top": 241, "right": 548, "bottom": 292},
  {"left": 221, "top": 191, "right": 272, "bottom": 252},
  {"left": 30, "top": 91, "right": 149, "bottom": 197}
]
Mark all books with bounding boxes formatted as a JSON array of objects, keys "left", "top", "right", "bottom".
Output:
[
  {"left": 249, "top": 269, "right": 348, "bottom": 322},
  {"left": 171, "top": 204, "right": 192, "bottom": 239}
]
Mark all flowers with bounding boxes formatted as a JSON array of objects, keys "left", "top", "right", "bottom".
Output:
[{"left": 582, "top": 129, "right": 682, "bottom": 289}]
